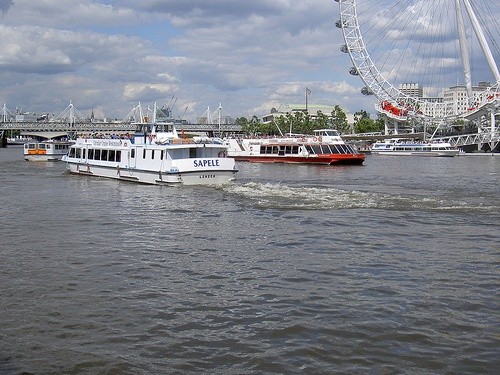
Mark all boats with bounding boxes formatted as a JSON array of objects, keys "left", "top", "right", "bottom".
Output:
[
  {"left": 367, "top": 141, "right": 460, "bottom": 159},
  {"left": 61, "top": 123, "right": 239, "bottom": 185},
  {"left": 21, "top": 138, "right": 74, "bottom": 161},
  {"left": 224, "top": 134, "right": 368, "bottom": 167}
]
